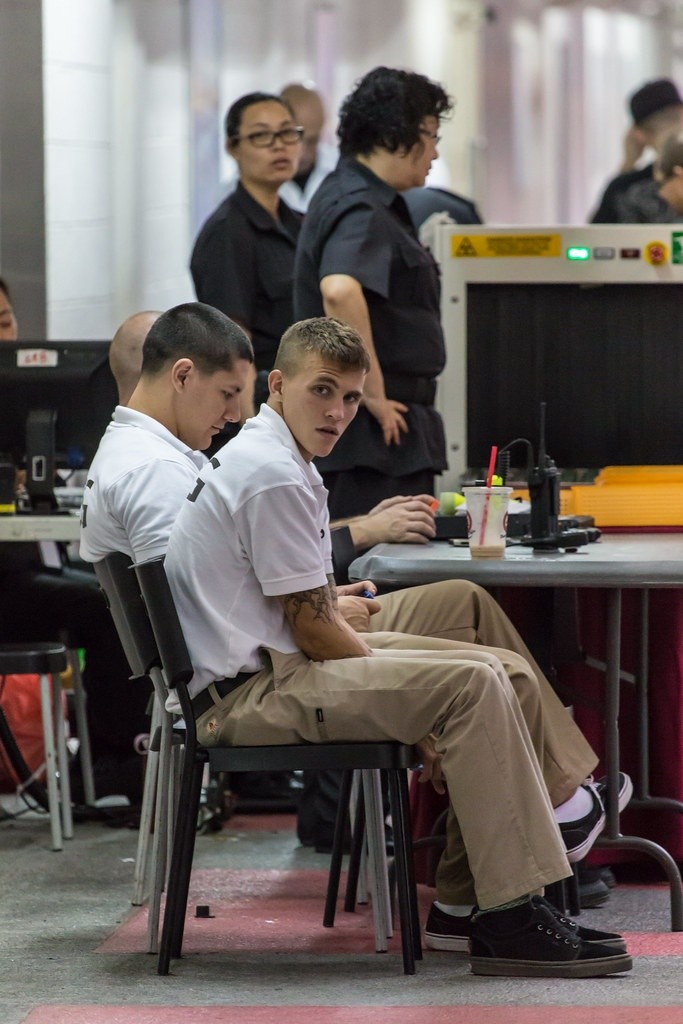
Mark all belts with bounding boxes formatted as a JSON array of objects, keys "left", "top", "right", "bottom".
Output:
[{"left": 189, "top": 671, "right": 256, "bottom": 720}]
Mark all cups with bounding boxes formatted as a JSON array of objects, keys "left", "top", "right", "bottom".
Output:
[{"left": 462, "top": 487, "right": 515, "bottom": 559}]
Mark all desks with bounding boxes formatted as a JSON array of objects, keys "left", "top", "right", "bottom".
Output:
[
  {"left": 0, "top": 507, "right": 82, "bottom": 567},
  {"left": 346, "top": 530, "right": 683, "bottom": 933}
]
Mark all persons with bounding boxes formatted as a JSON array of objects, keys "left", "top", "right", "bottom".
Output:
[
  {"left": 191, "top": 92, "right": 305, "bottom": 364},
  {"left": 110, "top": 309, "right": 436, "bottom": 568},
  {"left": 0, "top": 278, "right": 18, "bottom": 340},
  {"left": 277, "top": 84, "right": 339, "bottom": 215},
  {"left": 79, "top": 302, "right": 633, "bottom": 952},
  {"left": 163, "top": 318, "right": 633, "bottom": 977},
  {"left": 293, "top": 67, "right": 453, "bottom": 854},
  {"left": 594, "top": 79, "right": 683, "bottom": 224}
]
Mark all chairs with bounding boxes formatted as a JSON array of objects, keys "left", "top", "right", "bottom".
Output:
[
  {"left": 128, "top": 552, "right": 423, "bottom": 979},
  {"left": 1, "top": 642, "right": 76, "bottom": 852},
  {"left": 90, "top": 551, "right": 394, "bottom": 956}
]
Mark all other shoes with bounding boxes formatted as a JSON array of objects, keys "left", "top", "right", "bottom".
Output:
[
  {"left": 577, "top": 864, "right": 616, "bottom": 887},
  {"left": 545, "top": 877, "right": 610, "bottom": 907},
  {"left": 316, "top": 825, "right": 394, "bottom": 855}
]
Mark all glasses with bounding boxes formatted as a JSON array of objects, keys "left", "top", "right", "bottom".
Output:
[
  {"left": 234, "top": 127, "right": 304, "bottom": 148},
  {"left": 417, "top": 129, "right": 441, "bottom": 150}
]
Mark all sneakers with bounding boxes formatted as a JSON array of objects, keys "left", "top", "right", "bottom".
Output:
[
  {"left": 422, "top": 902, "right": 480, "bottom": 951},
  {"left": 558, "top": 769, "right": 633, "bottom": 861},
  {"left": 468, "top": 908, "right": 632, "bottom": 976},
  {"left": 531, "top": 894, "right": 627, "bottom": 950}
]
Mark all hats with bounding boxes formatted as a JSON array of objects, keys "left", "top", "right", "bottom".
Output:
[{"left": 631, "top": 81, "right": 680, "bottom": 124}]
